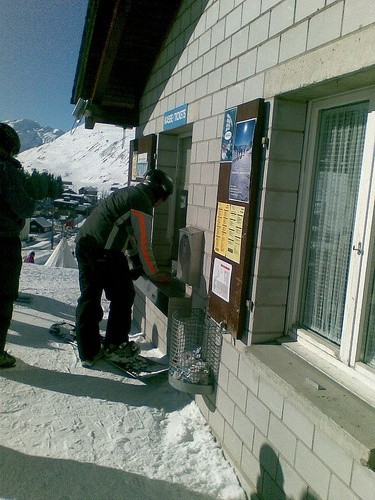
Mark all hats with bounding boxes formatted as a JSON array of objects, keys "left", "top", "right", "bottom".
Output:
[{"left": 1, "top": 122, "right": 21, "bottom": 158}]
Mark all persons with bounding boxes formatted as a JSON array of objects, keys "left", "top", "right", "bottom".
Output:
[
  {"left": 0, "top": 123, "right": 35, "bottom": 370},
  {"left": 69, "top": 169, "right": 176, "bottom": 366}
]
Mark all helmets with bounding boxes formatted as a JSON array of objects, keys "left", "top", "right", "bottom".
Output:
[{"left": 142, "top": 169, "right": 173, "bottom": 201}]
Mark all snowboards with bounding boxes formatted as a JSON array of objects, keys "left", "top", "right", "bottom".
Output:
[{"left": 49, "top": 322, "right": 169, "bottom": 380}]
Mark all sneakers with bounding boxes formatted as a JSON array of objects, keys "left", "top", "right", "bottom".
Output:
[
  {"left": 81, "top": 345, "right": 106, "bottom": 367},
  {"left": 0, "top": 351, "right": 16, "bottom": 367},
  {"left": 104, "top": 341, "right": 141, "bottom": 361}
]
